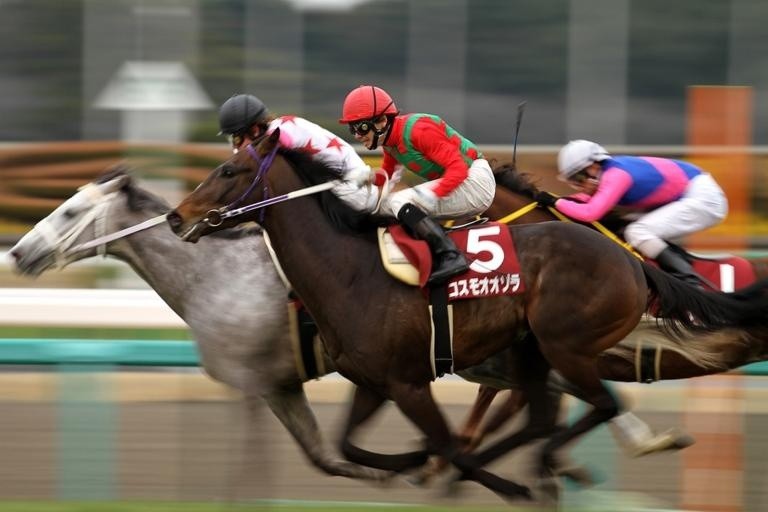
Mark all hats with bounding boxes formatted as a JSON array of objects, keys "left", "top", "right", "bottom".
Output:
[
  {"left": 557, "top": 139, "right": 613, "bottom": 178},
  {"left": 215, "top": 93, "right": 269, "bottom": 136},
  {"left": 340, "top": 84, "right": 399, "bottom": 125}
]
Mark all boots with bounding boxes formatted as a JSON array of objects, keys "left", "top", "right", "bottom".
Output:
[
  {"left": 657, "top": 245, "right": 703, "bottom": 297},
  {"left": 414, "top": 215, "right": 469, "bottom": 278}
]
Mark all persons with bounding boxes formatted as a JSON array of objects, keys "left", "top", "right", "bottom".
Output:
[
  {"left": 339, "top": 85, "right": 496, "bottom": 288},
  {"left": 536, "top": 140, "right": 729, "bottom": 323},
  {"left": 217, "top": 94, "right": 379, "bottom": 212}
]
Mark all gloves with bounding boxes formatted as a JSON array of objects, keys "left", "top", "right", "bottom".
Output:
[
  {"left": 346, "top": 166, "right": 372, "bottom": 185},
  {"left": 536, "top": 190, "right": 561, "bottom": 207}
]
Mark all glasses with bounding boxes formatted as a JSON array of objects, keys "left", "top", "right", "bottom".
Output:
[
  {"left": 231, "top": 129, "right": 248, "bottom": 147},
  {"left": 347, "top": 122, "right": 374, "bottom": 135}
]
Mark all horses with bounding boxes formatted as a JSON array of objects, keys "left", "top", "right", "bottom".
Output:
[{"left": 8, "top": 126, "right": 768, "bottom": 512}]
